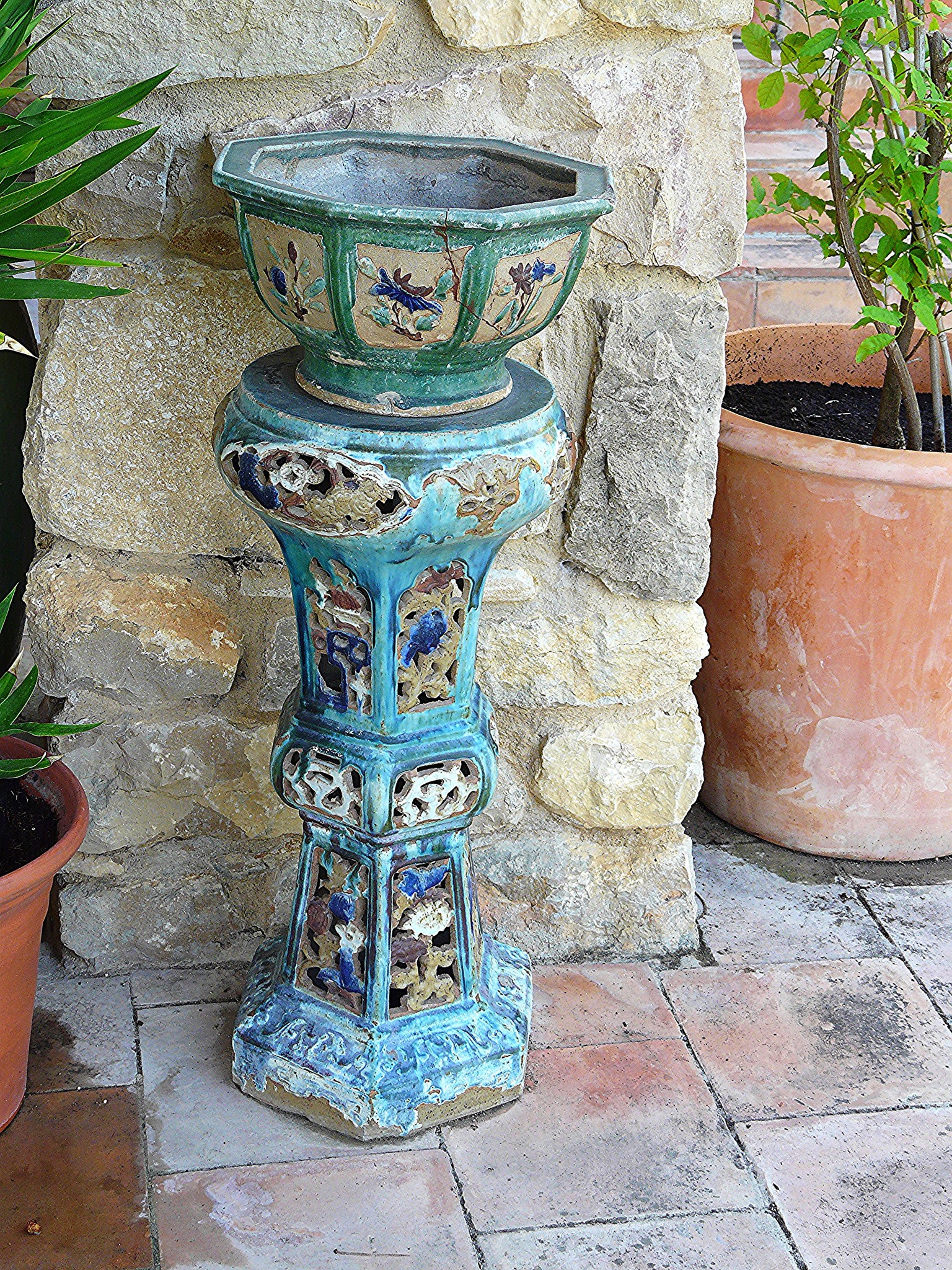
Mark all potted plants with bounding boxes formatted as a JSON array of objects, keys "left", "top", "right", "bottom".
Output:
[
  {"left": 702, "top": 0, "right": 952, "bottom": 862},
  {"left": 0, "top": 581, "right": 102, "bottom": 1133}
]
